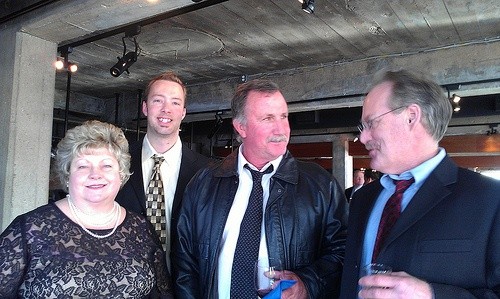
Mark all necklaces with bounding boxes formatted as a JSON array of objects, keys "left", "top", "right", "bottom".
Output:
[{"left": 66, "top": 194, "right": 122, "bottom": 239}]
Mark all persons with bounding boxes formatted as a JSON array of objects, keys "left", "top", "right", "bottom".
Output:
[
  {"left": 0, "top": 120, "right": 168, "bottom": 299},
  {"left": 263, "top": 67, "right": 500, "bottom": 299},
  {"left": 363, "top": 168, "right": 380, "bottom": 185},
  {"left": 345, "top": 169, "right": 365, "bottom": 202},
  {"left": 168, "top": 79, "right": 350, "bottom": 299},
  {"left": 114, "top": 72, "right": 221, "bottom": 299}
]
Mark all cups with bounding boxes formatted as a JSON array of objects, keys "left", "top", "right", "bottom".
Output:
[
  {"left": 363, "top": 264, "right": 392, "bottom": 289},
  {"left": 254, "top": 258, "right": 283, "bottom": 299}
]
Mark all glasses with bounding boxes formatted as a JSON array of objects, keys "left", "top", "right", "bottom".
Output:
[{"left": 358, "top": 105, "right": 414, "bottom": 132}]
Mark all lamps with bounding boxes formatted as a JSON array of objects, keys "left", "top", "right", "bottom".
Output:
[
  {"left": 298, "top": 0, "right": 316, "bottom": 14},
  {"left": 445, "top": 88, "right": 461, "bottom": 112},
  {"left": 110, "top": 36, "right": 141, "bottom": 77},
  {"left": 55, "top": 46, "right": 77, "bottom": 73},
  {"left": 207, "top": 111, "right": 224, "bottom": 140}
]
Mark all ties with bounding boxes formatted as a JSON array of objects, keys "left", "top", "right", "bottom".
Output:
[
  {"left": 146, "top": 154, "right": 169, "bottom": 252},
  {"left": 372, "top": 176, "right": 415, "bottom": 270},
  {"left": 230, "top": 163, "right": 275, "bottom": 299}
]
